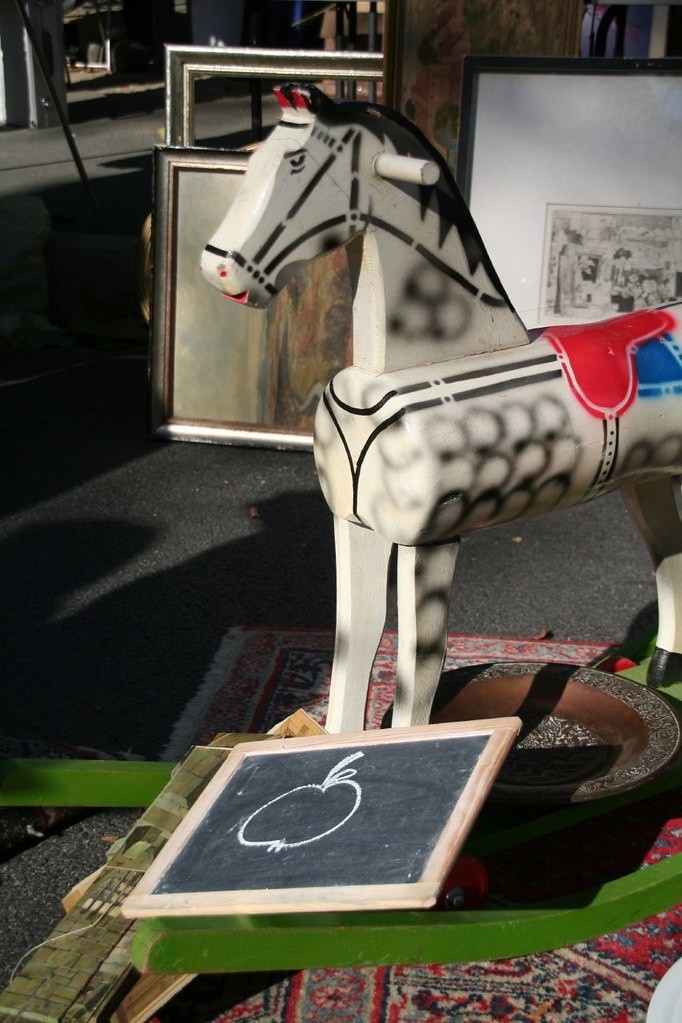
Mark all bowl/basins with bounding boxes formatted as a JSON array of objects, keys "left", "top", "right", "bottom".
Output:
[{"left": 381, "top": 660, "right": 681, "bottom": 805}]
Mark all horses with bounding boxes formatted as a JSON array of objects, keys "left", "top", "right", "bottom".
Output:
[{"left": 196, "top": 78, "right": 682, "bottom": 737}]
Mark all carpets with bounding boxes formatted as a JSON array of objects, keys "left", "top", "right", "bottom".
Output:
[{"left": 156, "top": 624, "right": 682, "bottom": 1023}]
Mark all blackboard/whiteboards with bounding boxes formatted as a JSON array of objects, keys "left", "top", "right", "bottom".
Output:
[{"left": 120, "top": 715, "right": 524, "bottom": 920}]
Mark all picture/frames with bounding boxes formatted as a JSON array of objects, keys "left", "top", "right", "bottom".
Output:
[
  {"left": 121, "top": 713, "right": 525, "bottom": 916},
  {"left": 454, "top": 55, "right": 682, "bottom": 332},
  {"left": 149, "top": 145, "right": 354, "bottom": 453}
]
